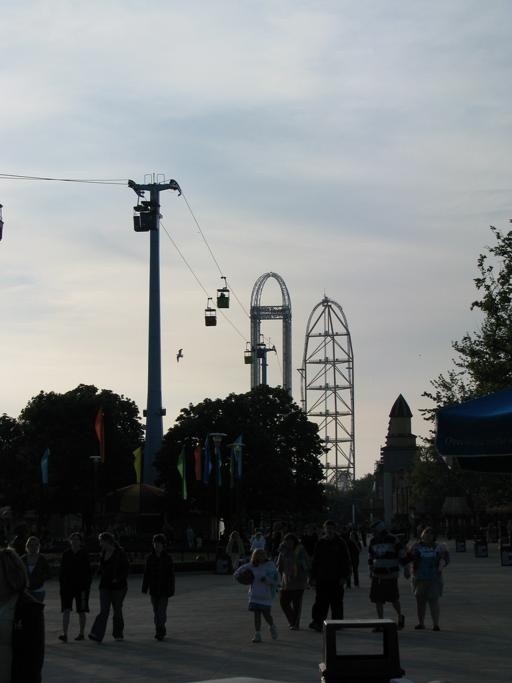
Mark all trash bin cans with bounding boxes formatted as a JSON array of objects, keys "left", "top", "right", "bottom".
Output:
[
  {"left": 499, "top": 537, "right": 511, "bottom": 564},
  {"left": 474, "top": 534, "right": 487, "bottom": 557},
  {"left": 318, "top": 619, "right": 406, "bottom": 682},
  {"left": 455, "top": 533, "right": 466, "bottom": 552}
]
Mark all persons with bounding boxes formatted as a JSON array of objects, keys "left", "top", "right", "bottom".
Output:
[
  {"left": 1, "top": 516, "right": 225, "bottom": 554},
  {"left": 306, "top": 521, "right": 349, "bottom": 631},
  {"left": 58, "top": 533, "right": 93, "bottom": 643},
  {"left": 227, "top": 512, "right": 362, "bottom": 591},
  {"left": 234, "top": 549, "right": 279, "bottom": 642},
  {"left": 365, "top": 520, "right": 406, "bottom": 631},
  {"left": 1, "top": 535, "right": 48, "bottom": 683},
  {"left": 89, "top": 531, "right": 129, "bottom": 641},
  {"left": 400, "top": 527, "right": 450, "bottom": 630},
  {"left": 276, "top": 533, "right": 311, "bottom": 629},
  {"left": 360, "top": 512, "right": 435, "bottom": 546},
  {"left": 141, "top": 535, "right": 175, "bottom": 640}
]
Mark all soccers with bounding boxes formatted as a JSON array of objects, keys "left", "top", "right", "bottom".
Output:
[{"left": 238, "top": 569, "right": 253, "bottom": 585}]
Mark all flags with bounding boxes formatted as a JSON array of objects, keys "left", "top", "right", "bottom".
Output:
[
  {"left": 41, "top": 448, "right": 49, "bottom": 488},
  {"left": 175, "top": 432, "right": 243, "bottom": 498},
  {"left": 131, "top": 460, "right": 133, "bottom": 461},
  {"left": 94, "top": 408, "right": 105, "bottom": 463},
  {"left": 132, "top": 446, "right": 141, "bottom": 487}
]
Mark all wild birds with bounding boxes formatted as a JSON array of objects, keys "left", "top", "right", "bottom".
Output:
[{"left": 176, "top": 347, "right": 183, "bottom": 362}]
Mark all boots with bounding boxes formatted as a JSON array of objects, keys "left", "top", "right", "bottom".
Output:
[
  {"left": 252, "top": 631, "right": 261, "bottom": 642},
  {"left": 269, "top": 625, "right": 277, "bottom": 639}
]
